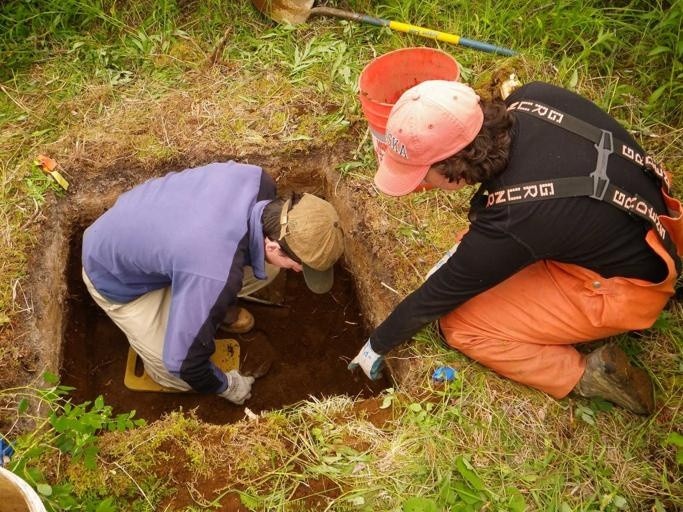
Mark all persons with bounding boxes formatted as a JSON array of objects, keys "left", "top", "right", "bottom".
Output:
[
  {"left": 78, "top": 160, "right": 347, "bottom": 408},
  {"left": 344, "top": 76, "right": 681, "bottom": 418}
]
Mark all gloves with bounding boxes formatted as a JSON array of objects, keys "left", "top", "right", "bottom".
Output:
[
  {"left": 348, "top": 338, "right": 383, "bottom": 381},
  {"left": 217, "top": 369, "right": 255, "bottom": 405}
]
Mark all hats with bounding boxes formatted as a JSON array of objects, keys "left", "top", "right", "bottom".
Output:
[
  {"left": 279, "top": 192, "right": 345, "bottom": 294},
  {"left": 374, "top": 80, "right": 484, "bottom": 197}
]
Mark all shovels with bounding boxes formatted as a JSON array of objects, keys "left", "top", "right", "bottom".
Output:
[{"left": 249, "top": 0, "right": 518, "bottom": 57}]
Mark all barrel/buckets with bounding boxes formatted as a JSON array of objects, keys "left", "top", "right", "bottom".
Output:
[
  {"left": 356, "top": 47, "right": 461, "bottom": 193},
  {"left": 0, "top": 467, "right": 49, "bottom": 512}
]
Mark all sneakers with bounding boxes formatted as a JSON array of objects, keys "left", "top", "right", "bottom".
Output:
[
  {"left": 574, "top": 343, "right": 654, "bottom": 415},
  {"left": 219, "top": 306, "right": 254, "bottom": 332}
]
